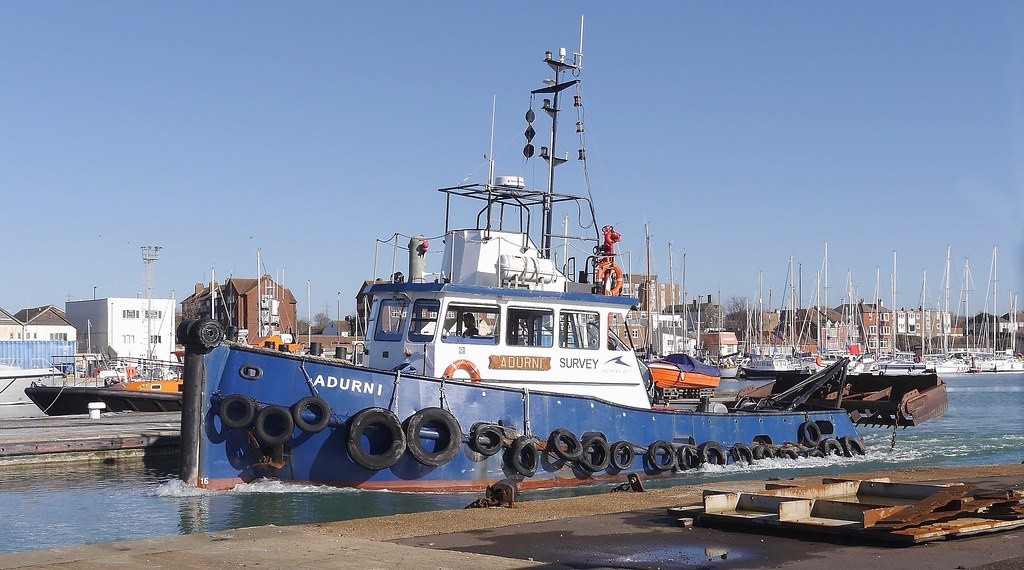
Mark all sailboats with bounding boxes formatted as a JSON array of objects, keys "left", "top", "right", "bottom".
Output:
[{"left": 23, "top": 213, "right": 1024, "bottom": 419}]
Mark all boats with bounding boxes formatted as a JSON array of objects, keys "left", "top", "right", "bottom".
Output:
[
  {"left": 646, "top": 354, "right": 723, "bottom": 389},
  {"left": 180, "top": 13, "right": 949, "bottom": 493}
]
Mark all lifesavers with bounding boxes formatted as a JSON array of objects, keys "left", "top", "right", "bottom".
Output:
[
  {"left": 507, "top": 435, "right": 539, "bottom": 478},
  {"left": 406, "top": 407, "right": 462, "bottom": 467},
  {"left": 731, "top": 436, "right": 865, "bottom": 467},
  {"left": 612, "top": 441, "right": 635, "bottom": 470},
  {"left": 584, "top": 436, "right": 612, "bottom": 473},
  {"left": 678, "top": 446, "right": 700, "bottom": 471},
  {"left": 798, "top": 421, "right": 821, "bottom": 447},
  {"left": 344, "top": 407, "right": 406, "bottom": 470},
  {"left": 127, "top": 366, "right": 137, "bottom": 378},
  {"left": 596, "top": 261, "right": 624, "bottom": 296},
  {"left": 219, "top": 393, "right": 255, "bottom": 429},
  {"left": 648, "top": 440, "right": 676, "bottom": 472},
  {"left": 470, "top": 422, "right": 504, "bottom": 456},
  {"left": 294, "top": 396, "right": 332, "bottom": 433},
  {"left": 700, "top": 441, "right": 728, "bottom": 471},
  {"left": 549, "top": 428, "right": 584, "bottom": 462},
  {"left": 444, "top": 360, "right": 482, "bottom": 384},
  {"left": 255, "top": 405, "right": 294, "bottom": 446}
]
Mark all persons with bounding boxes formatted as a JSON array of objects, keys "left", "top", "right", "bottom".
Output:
[{"left": 462, "top": 313, "right": 479, "bottom": 337}]
